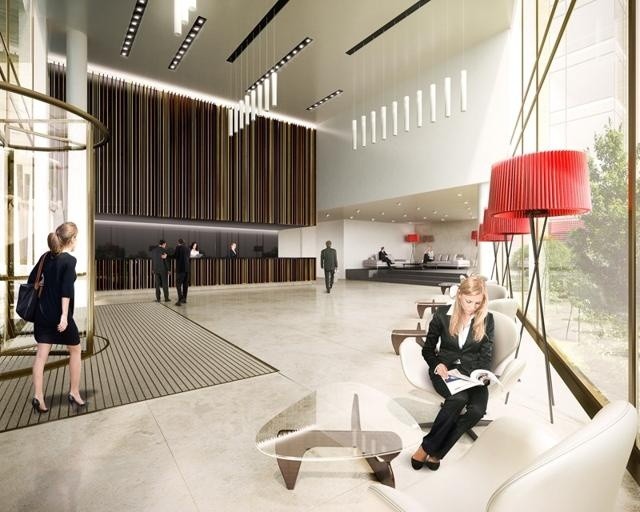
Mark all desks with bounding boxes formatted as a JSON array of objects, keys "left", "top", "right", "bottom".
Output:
[
  {"left": 438, "top": 281, "right": 459, "bottom": 296},
  {"left": 391, "top": 321, "right": 427, "bottom": 355},
  {"left": 415, "top": 297, "right": 452, "bottom": 320}
]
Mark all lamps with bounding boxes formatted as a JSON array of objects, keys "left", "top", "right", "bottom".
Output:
[
  {"left": 173, "top": 0, "right": 196, "bottom": 37},
  {"left": 227, "top": 0, "right": 277, "bottom": 137},
  {"left": 407, "top": 234, "right": 419, "bottom": 262},
  {"left": 346, "top": 0, "right": 467, "bottom": 150},
  {"left": 471, "top": 150, "right": 591, "bottom": 424}
]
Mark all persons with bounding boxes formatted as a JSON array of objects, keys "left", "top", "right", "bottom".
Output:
[
  {"left": 379, "top": 246, "right": 395, "bottom": 269},
  {"left": 189, "top": 241, "right": 200, "bottom": 258},
  {"left": 230, "top": 242, "right": 237, "bottom": 257},
  {"left": 410, "top": 274, "right": 494, "bottom": 471},
  {"left": 25, "top": 221, "right": 90, "bottom": 414},
  {"left": 320, "top": 240, "right": 338, "bottom": 293},
  {"left": 152, "top": 239, "right": 172, "bottom": 302},
  {"left": 423, "top": 246, "right": 434, "bottom": 264},
  {"left": 161, "top": 238, "right": 190, "bottom": 307}
]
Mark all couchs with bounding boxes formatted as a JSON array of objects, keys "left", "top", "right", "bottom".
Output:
[
  {"left": 416, "top": 258, "right": 470, "bottom": 269},
  {"left": 363, "top": 258, "right": 409, "bottom": 270}
]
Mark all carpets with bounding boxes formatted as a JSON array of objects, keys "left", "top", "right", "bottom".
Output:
[{"left": 0, "top": 302, "right": 279, "bottom": 433}]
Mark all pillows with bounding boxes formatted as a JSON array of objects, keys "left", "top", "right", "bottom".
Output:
[
  {"left": 368, "top": 255, "right": 392, "bottom": 260},
  {"left": 434, "top": 254, "right": 463, "bottom": 261}
]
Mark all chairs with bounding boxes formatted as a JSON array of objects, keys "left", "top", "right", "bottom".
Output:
[
  {"left": 485, "top": 285, "right": 509, "bottom": 302},
  {"left": 486, "top": 297, "right": 520, "bottom": 322},
  {"left": 369, "top": 396, "right": 640, "bottom": 512},
  {"left": 459, "top": 275, "right": 499, "bottom": 285},
  {"left": 398, "top": 310, "right": 526, "bottom": 442}
]
